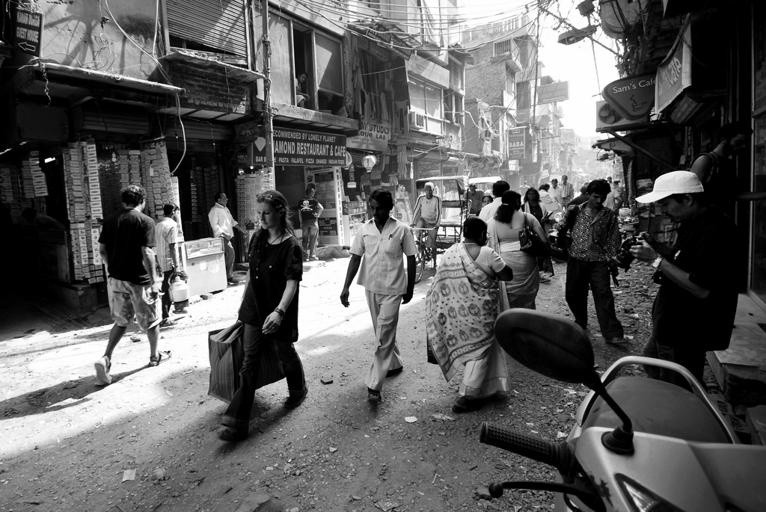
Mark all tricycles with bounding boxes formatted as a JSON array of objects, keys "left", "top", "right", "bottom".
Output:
[
  {"left": 463, "top": 177, "right": 502, "bottom": 222},
  {"left": 410, "top": 175, "right": 471, "bottom": 285}
]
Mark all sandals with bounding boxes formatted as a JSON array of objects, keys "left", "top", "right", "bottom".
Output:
[
  {"left": 94, "top": 358, "right": 112, "bottom": 384},
  {"left": 149, "top": 351, "right": 172, "bottom": 365}
]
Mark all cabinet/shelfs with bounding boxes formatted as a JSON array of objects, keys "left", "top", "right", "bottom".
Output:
[
  {"left": 185, "top": 237, "right": 228, "bottom": 296},
  {"left": 344, "top": 212, "right": 369, "bottom": 246}
]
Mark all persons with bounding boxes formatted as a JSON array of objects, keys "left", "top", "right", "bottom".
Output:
[
  {"left": 558, "top": 175, "right": 575, "bottom": 208},
  {"left": 520, "top": 180, "right": 529, "bottom": 195},
  {"left": 689, "top": 120, "right": 753, "bottom": 209},
  {"left": 94, "top": 185, "right": 172, "bottom": 384},
  {"left": 629, "top": 171, "right": 738, "bottom": 394},
  {"left": 339, "top": 188, "right": 418, "bottom": 402},
  {"left": 218, "top": 189, "right": 308, "bottom": 443},
  {"left": 549, "top": 179, "right": 562, "bottom": 204},
  {"left": 534, "top": 183, "right": 563, "bottom": 220},
  {"left": 462, "top": 184, "right": 485, "bottom": 218},
  {"left": 422, "top": 218, "right": 513, "bottom": 413},
  {"left": 296, "top": 189, "right": 324, "bottom": 261},
  {"left": 409, "top": 182, "right": 442, "bottom": 271},
  {"left": 155, "top": 202, "right": 189, "bottom": 327},
  {"left": 292, "top": 71, "right": 310, "bottom": 107},
  {"left": 476, "top": 196, "right": 494, "bottom": 217},
  {"left": 605, "top": 180, "right": 623, "bottom": 212},
  {"left": 566, "top": 182, "right": 592, "bottom": 207},
  {"left": 556, "top": 179, "right": 628, "bottom": 345},
  {"left": 209, "top": 191, "right": 248, "bottom": 284},
  {"left": 479, "top": 180, "right": 511, "bottom": 236},
  {"left": 487, "top": 191, "right": 548, "bottom": 311},
  {"left": 519, "top": 187, "right": 555, "bottom": 282},
  {"left": 22, "top": 206, "right": 68, "bottom": 237},
  {"left": 605, "top": 176, "right": 616, "bottom": 191}
]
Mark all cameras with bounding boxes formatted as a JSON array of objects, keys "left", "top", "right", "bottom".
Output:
[{"left": 611, "top": 236, "right": 643, "bottom": 271}]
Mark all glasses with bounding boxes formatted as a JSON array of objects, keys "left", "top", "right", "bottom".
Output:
[{"left": 256, "top": 193, "right": 273, "bottom": 200}]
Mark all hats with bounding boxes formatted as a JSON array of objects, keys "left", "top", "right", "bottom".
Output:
[{"left": 634, "top": 170, "right": 703, "bottom": 205}]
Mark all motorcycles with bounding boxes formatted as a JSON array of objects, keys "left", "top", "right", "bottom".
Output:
[{"left": 478, "top": 308, "right": 766, "bottom": 512}]
